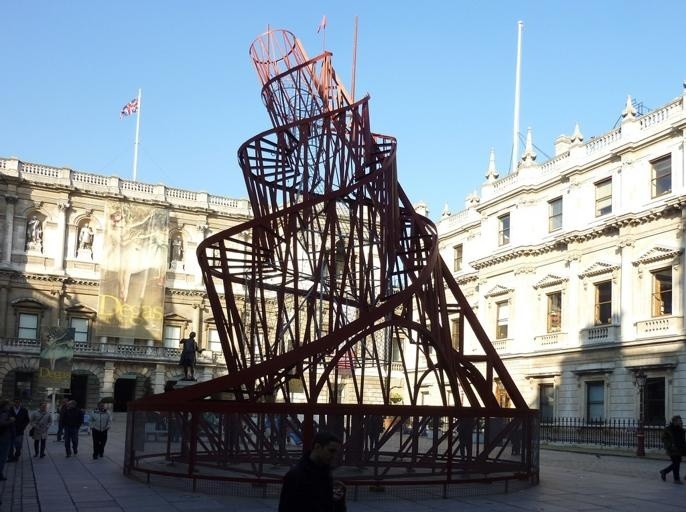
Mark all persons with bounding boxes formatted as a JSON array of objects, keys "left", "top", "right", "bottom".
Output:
[
  {"left": 279, "top": 431, "right": 349, "bottom": 512},
  {"left": 86, "top": 402, "right": 110, "bottom": 461},
  {"left": 659, "top": 416, "right": 686, "bottom": 483},
  {"left": 79, "top": 222, "right": 94, "bottom": 252},
  {"left": 28, "top": 215, "right": 42, "bottom": 243},
  {"left": 180, "top": 331, "right": 205, "bottom": 378},
  {"left": 0, "top": 395, "right": 33, "bottom": 480},
  {"left": 172, "top": 235, "right": 182, "bottom": 261},
  {"left": 55, "top": 395, "right": 83, "bottom": 456},
  {"left": 28, "top": 402, "right": 53, "bottom": 459}
]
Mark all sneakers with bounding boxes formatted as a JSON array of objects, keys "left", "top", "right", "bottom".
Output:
[
  {"left": 7, "top": 437, "right": 104, "bottom": 464},
  {"left": 660, "top": 469, "right": 666, "bottom": 482}
]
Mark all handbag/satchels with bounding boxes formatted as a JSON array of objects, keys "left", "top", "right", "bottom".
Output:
[{"left": 29, "top": 427, "right": 35, "bottom": 436}]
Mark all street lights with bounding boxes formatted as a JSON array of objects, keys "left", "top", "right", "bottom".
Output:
[{"left": 636, "top": 368, "right": 647, "bottom": 456}]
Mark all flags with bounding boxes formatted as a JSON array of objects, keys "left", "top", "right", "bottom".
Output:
[{"left": 120, "top": 100, "right": 139, "bottom": 121}]
